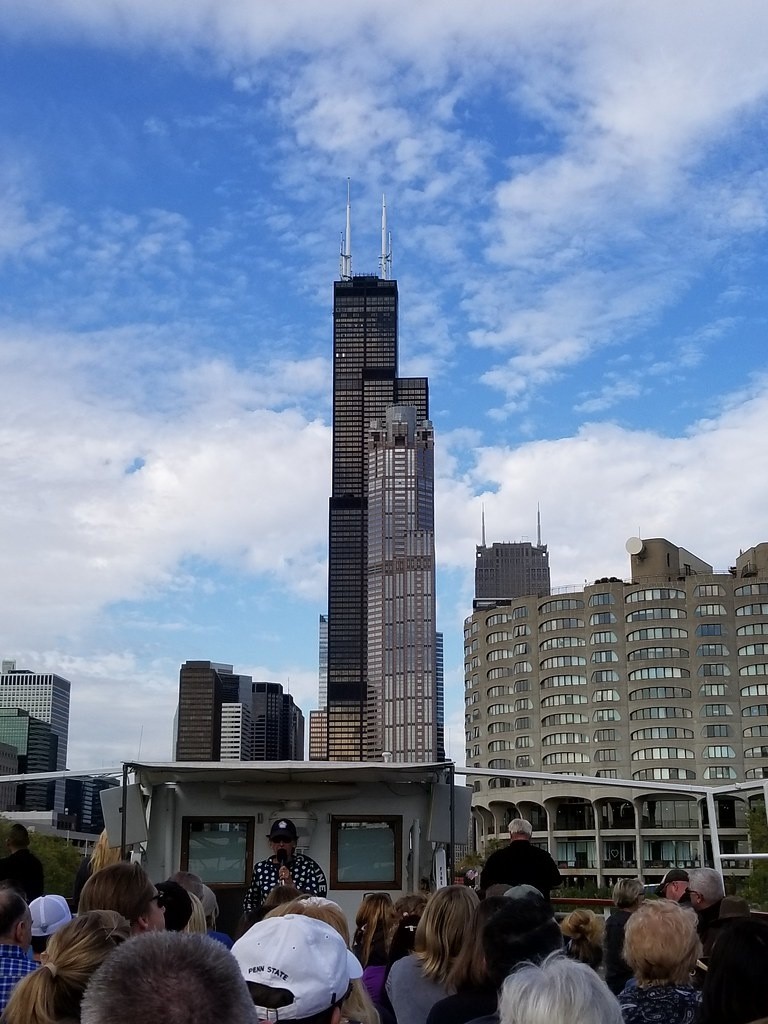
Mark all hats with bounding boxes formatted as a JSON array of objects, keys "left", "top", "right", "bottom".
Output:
[
  {"left": 718, "top": 896, "right": 751, "bottom": 918},
  {"left": 229, "top": 913, "right": 364, "bottom": 1023},
  {"left": 268, "top": 817, "right": 297, "bottom": 839},
  {"left": 154, "top": 881, "right": 192, "bottom": 930},
  {"left": 28, "top": 894, "right": 72, "bottom": 937},
  {"left": 655, "top": 870, "right": 690, "bottom": 892}
]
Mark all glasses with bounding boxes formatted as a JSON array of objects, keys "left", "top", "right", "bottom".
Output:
[
  {"left": 363, "top": 893, "right": 391, "bottom": 901},
  {"left": 146, "top": 891, "right": 164, "bottom": 908},
  {"left": 685, "top": 887, "right": 703, "bottom": 897},
  {"left": 272, "top": 837, "right": 295, "bottom": 844}
]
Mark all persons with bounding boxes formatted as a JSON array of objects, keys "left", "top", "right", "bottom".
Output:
[
  {"left": 0, "top": 824, "right": 44, "bottom": 900},
  {"left": 479, "top": 819, "right": 561, "bottom": 902},
  {"left": 72, "top": 828, "right": 129, "bottom": 912},
  {"left": 243, "top": 818, "right": 327, "bottom": 922},
  {"left": 0, "top": 867, "right": 768, "bottom": 1024}
]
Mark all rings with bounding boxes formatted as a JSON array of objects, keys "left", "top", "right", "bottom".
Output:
[{"left": 283, "top": 872, "right": 284, "bottom": 875}]
